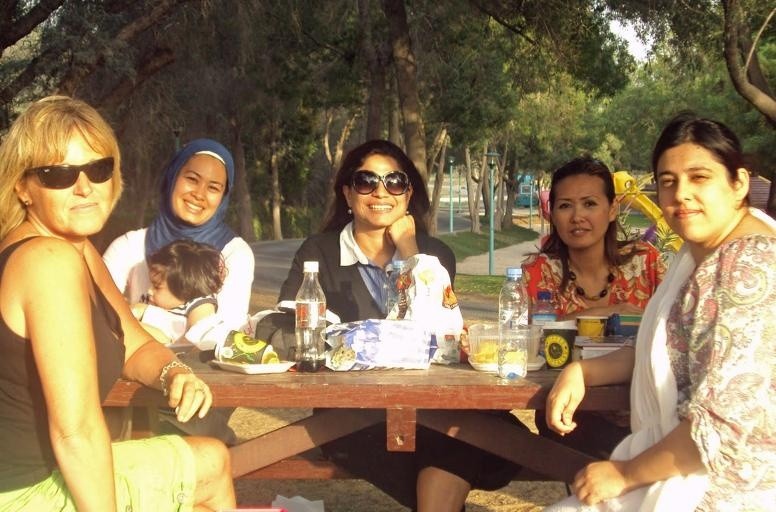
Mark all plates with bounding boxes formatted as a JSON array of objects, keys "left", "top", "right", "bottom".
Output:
[{"left": 210, "top": 358, "right": 296, "bottom": 373}]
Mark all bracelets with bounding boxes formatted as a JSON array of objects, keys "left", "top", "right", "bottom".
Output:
[{"left": 160, "top": 361, "right": 194, "bottom": 397}]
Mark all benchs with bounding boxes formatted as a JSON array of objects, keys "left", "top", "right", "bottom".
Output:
[{"left": 115, "top": 429, "right": 363, "bottom": 481}]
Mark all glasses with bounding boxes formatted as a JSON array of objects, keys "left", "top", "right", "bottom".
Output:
[
  {"left": 350, "top": 170, "right": 410, "bottom": 196},
  {"left": 23, "top": 155, "right": 114, "bottom": 190}
]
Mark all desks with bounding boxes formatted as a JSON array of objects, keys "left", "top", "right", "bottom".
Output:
[{"left": 101, "top": 342, "right": 630, "bottom": 451}]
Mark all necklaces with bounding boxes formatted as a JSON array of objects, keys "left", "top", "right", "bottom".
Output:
[{"left": 566, "top": 259, "right": 617, "bottom": 300}]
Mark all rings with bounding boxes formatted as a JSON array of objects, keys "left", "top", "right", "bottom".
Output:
[{"left": 194, "top": 383, "right": 208, "bottom": 394}]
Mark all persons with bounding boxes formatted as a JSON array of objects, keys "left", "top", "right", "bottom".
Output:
[
  {"left": 128, "top": 237, "right": 229, "bottom": 347},
  {"left": 101, "top": 136, "right": 255, "bottom": 348},
  {"left": 277, "top": 140, "right": 530, "bottom": 512},
  {"left": 0, "top": 94, "right": 237, "bottom": 512},
  {"left": 515, "top": 151, "right": 668, "bottom": 461},
  {"left": 539, "top": 112, "right": 776, "bottom": 512}
]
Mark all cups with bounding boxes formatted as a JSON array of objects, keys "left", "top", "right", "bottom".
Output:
[
  {"left": 214, "top": 326, "right": 273, "bottom": 364},
  {"left": 543, "top": 321, "right": 577, "bottom": 370},
  {"left": 576, "top": 315, "right": 609, "bottom": 337}
]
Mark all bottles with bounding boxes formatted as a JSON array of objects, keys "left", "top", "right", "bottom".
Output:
[
  {"left": 532, "top": 290, "right": 559, "bottom": 352},
  {"left": 498, "top": 267, "right": 527, "bottom": 378},
  {"left": 387, "top": 260, "right": 417, "bottom": 321},
  {"left": 294, "top": 261, "right": 327, "bottom": 371}
]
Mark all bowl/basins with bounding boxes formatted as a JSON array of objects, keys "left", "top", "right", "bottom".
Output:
[{"left": 466, "top": 322, "right": 541, "bottom": 373}]
